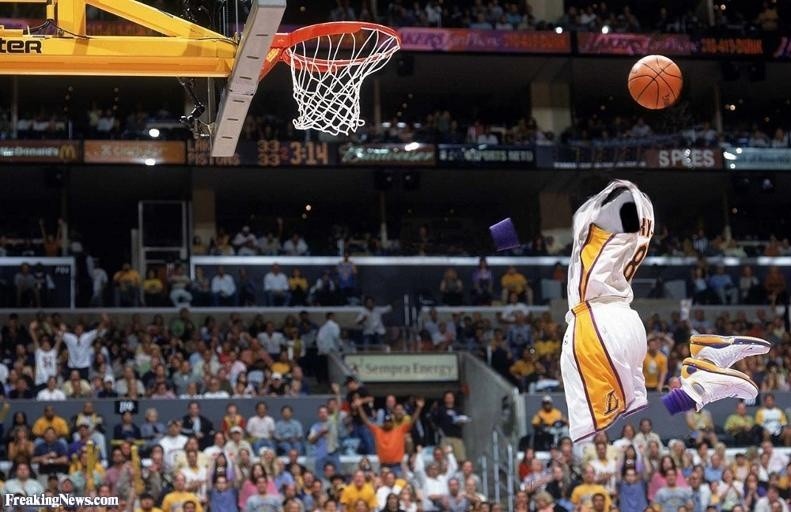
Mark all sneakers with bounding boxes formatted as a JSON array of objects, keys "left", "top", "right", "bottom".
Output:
[
  {"left": 688, "top": 333, "right": 772, "bottom": 369},
  {"left": 679, "top": 359, "right": 759, "bottom": 412}
]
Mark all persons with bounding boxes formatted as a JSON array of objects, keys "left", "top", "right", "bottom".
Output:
[{"left": 1, "top": 0, "right": 791, "bottom": 510}]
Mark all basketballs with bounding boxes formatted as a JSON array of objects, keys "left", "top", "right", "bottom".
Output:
[{"left": 629, "top": 55, "right": 684, "bottom": 110}]
